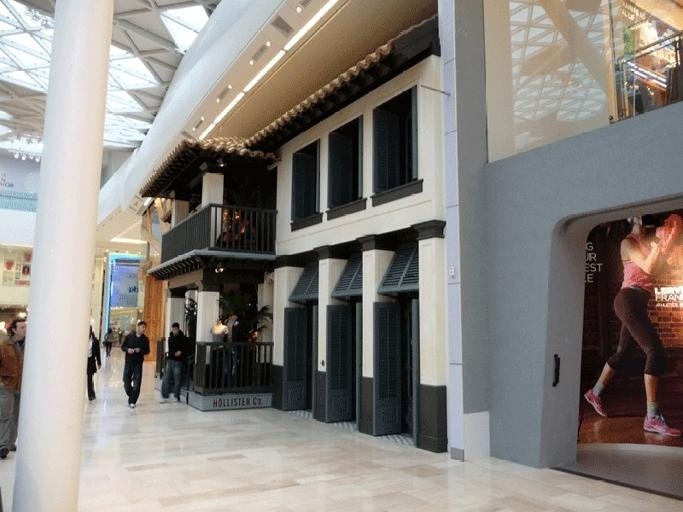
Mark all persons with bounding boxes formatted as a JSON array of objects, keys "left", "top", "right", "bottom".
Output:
[
  {"left": 208, "top": 320, "right": 228, "bottom": 388},
  {"left": 121, "top": 321, "right": 150, "bottom": 408},
  {"left": 112, "top": 326, "right": 131, "bottom": 344},
  {"left": 159, "top": 322, "right": 188, "bottom": 403},
  {"left": 104, "top": 328, "right": 113, "bottom": 357},
  {"left": 87, "top": 326, "right": 101, "bottom": 401},
  {"left": 0, "top": 317, "right": 27, "bottom": 457},
  {"left": 582, "top": 213, "right": 681, "bottom": 437}
]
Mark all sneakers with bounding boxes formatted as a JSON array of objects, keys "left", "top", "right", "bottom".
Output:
[
  {"left": 159, "top": 397, "right": 179, "bottom": 403},
  {"left": 584, "top": 389, "right": 608, "bottom": 417},
  {"left": 0, "top": 448, "right": 9, "bottom": 458},
  {"left": 643, "top": 413, "right": 683, "bottom": 437}
]
